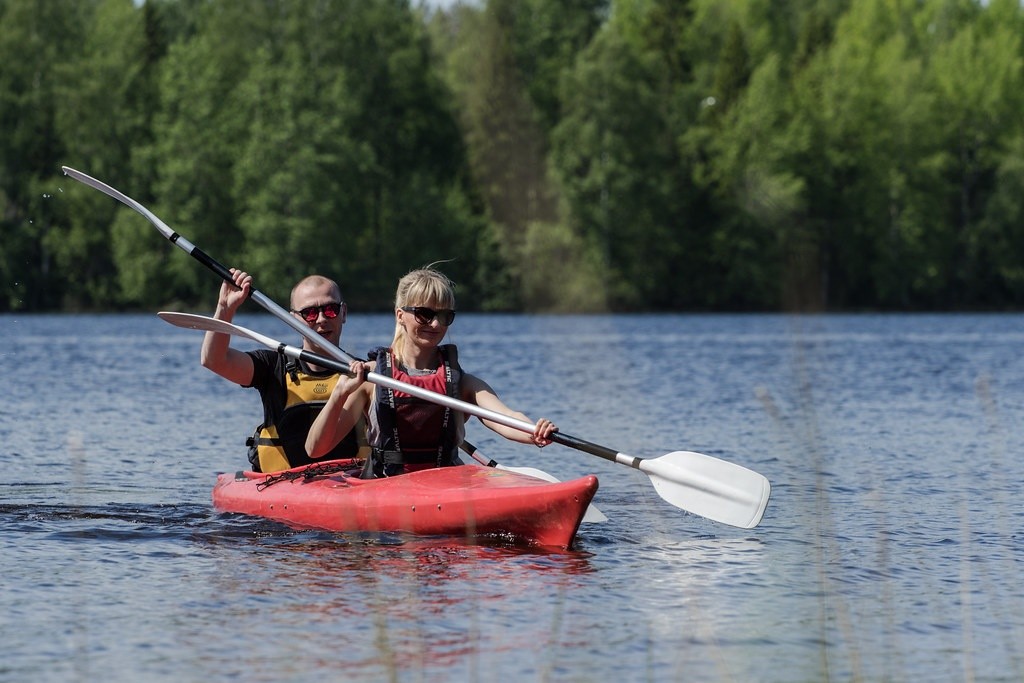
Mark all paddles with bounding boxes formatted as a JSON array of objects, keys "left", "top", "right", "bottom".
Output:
[
  {"left": 155, "top": 309, "right": 774, "bottom": 530},
  {"left": 60, "top": 165, "right": 610, "bottom": 523}
]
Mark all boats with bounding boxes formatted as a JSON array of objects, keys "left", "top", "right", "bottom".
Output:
[{"left": 212, "top": 458, "right": 600, "bottom": 556}]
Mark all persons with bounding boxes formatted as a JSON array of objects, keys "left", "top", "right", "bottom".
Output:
[
  {"left": 201, "top": 266, "right": 369, "bottom": 474},
  {"left": 305, "top": 266, "right": 560, "bottom": 481}
]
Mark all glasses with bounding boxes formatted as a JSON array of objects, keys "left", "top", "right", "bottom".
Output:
[
  {"left": 400, "top": 305, "right": 457, "bottom": 327},
  {"left": 290, "top": 302, "right": 344, "bottom": 322}
]
